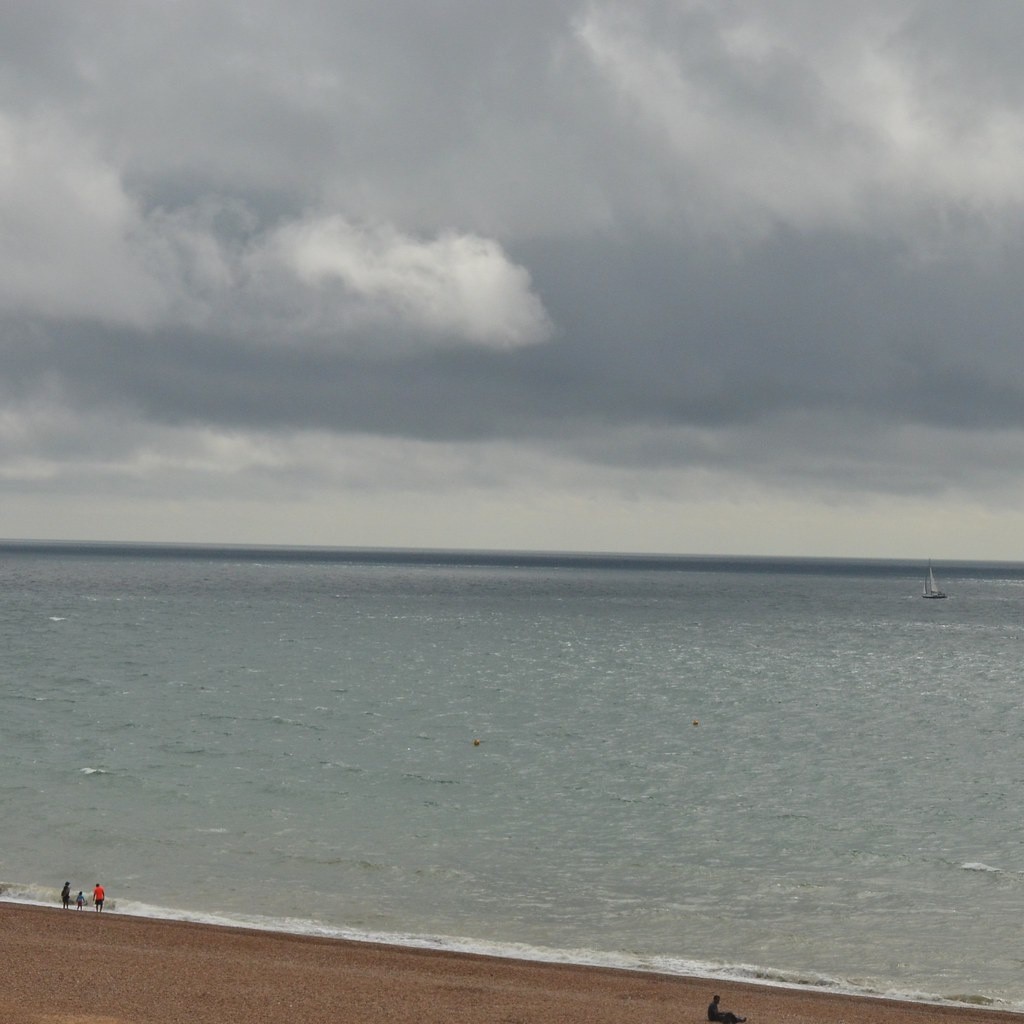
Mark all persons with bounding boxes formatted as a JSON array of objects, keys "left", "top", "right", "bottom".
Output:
[
  {"left": 61, "top": 882, "right": 70, "bottom": 910},
  {"left": 76, "top": 891, "right": 84, "bottom": 911},
  {"left": 708, "top": 995, "right": 747, "bottom": 1023},
  {"left": 93, "top": 884, "right": 105, "bottom": 912}
]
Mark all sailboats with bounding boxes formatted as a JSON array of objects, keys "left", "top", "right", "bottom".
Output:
[{"left": 921, "top": 558, "right": 948, "bottom": 598}]
